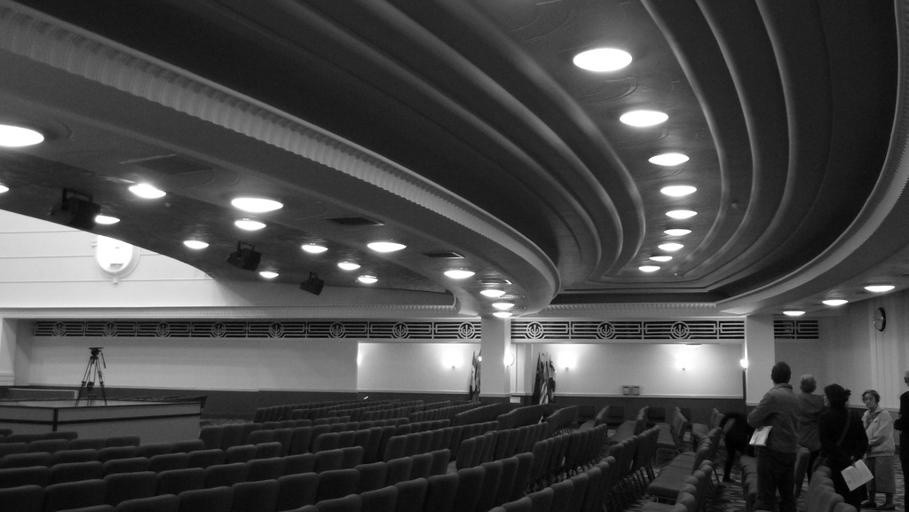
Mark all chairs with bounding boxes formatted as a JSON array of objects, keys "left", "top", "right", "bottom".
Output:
[{"left": 2, "top": 398, "right": 860, "bottom": 510}]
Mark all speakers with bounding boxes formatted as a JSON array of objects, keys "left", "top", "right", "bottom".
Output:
[
  {"left": 300, "top": 277, "right": 325, "bottom": 296},
  {"left": 49, "top": 196, "right": 100, "bottom": 230},
  {"left": 228, "top": 249, "right": 262, "bottom": 271}
]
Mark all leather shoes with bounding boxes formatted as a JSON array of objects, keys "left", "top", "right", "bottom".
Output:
[{"left": 723, "top": 475, "right": 737, "bottom": 484}]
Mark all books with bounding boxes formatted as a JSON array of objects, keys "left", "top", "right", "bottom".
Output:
[
  {"left": 749, "top": 425, "right": 774, "bottom": 447},
  {"left": 840, "top": 458, "right": 874, "bottom": 491}
]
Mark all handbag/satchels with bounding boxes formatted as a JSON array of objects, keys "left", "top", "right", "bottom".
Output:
[{"left": 810, "top": 457, "right": 834, "bottom": 476}]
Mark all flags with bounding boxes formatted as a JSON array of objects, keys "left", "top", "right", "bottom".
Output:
[
  {"left": 469, "top": 348, "right": 481, "bottom": 400},
  {"left": 530, "top": 351, "right": 556, "bottom": 405}
]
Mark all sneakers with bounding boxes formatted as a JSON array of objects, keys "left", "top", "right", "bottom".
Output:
[{"left": 860, "top": 501, "right": 895, "bottom": 510}]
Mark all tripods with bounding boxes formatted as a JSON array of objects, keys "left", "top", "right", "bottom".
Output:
[{"left": 75, "top": 355, "right": 107, "bottom": 407}]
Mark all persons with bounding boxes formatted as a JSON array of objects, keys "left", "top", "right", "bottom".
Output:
[
  {"left": 893, "top": 370, "right": 909, "bottom": 512},
  {"left": 860, "top": 390, "right": 897, "bottom": 510},
  {"left": 818, "top": 384, "right": 869, "bottom": 512},
  {"left": 718, "top": 411, "right": 755, "bottom": 483},
  {"left": 795, "top": 372, "right": 825, "bottom": 498},
  {"left": 745, "top": 360, "right": 800, "bottom": 512}
]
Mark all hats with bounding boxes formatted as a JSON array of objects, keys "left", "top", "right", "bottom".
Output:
[{"left": 825, "top": 383, "right": 851, "bottom": 407}]
